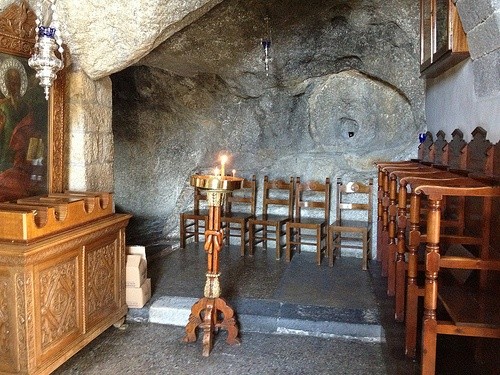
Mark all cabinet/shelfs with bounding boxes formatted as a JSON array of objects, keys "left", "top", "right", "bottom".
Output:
[
  {"left": 418, "top": 0, "right": 470, "bottom": 79},
  {"left": 0, "top": 212, "right": 134, "bottom": 375}
]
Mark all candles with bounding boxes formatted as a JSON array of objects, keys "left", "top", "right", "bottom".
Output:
[
  {"left": 221, "top": 154, "right": 227, "bottom": 179},
  {"left": 232, "top": 169, "right": 236, "bottom": 177},
  {"left": 213, "top": 167, "right": 220, "bottom": 179}
]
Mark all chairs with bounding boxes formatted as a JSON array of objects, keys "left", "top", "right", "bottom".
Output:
[
  {"left": 220, "top": 175, "right": 256, "bottom": 257},
  {"left": 249, "top": 175, "right": 294, "bottom": 261},
  {"left": 180, "top": 173, "right": 209, "bottom": 249},
  {"left": 286, "top": 176, "right": 331, "bottom": 265},
  {"left": 328, "top": 178, "right": 374, "bottom": 271}
]
